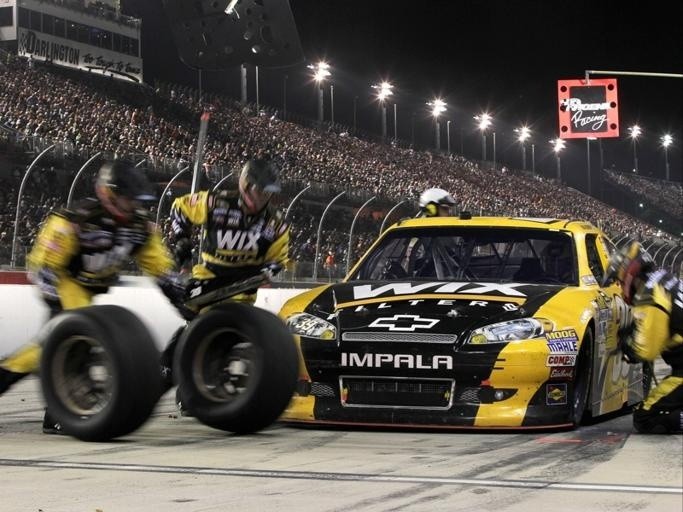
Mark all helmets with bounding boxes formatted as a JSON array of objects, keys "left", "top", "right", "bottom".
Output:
[
  {"left": 97, "top": 159, "right": 156, "bottom": 222},
  {"left": 418, "top": 187, "right": 456, "bottom": 216},
  {"left": 604, "top": 234, "right": 658, "bottom": 304},
  {"left": 239, "top": 157, "right": 280, "bottom": 212}
]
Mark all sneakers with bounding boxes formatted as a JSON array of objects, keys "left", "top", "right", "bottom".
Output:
[{"left": 41, "top": 406, "right": 68, "bottom": 434}]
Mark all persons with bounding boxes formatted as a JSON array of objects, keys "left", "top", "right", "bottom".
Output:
[
  {"left": 605, "top": 238, "right": 683, "bottom": 435},
  {"left": 538, "top": 243, "right": 570, "bottom": 281},
  {"left": 0, "top": 157, "right": 200, "bottom": 436},
  {"left": 404, "top": 188, "right": 457, "bottom": 276},
  {"left": 162, "top": 157, "right": 289, "bottom": 417}
]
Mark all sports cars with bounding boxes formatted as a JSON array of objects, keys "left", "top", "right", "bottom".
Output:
[{"left": 278, "top": 211, "right": 654, "bottom": 432}]
